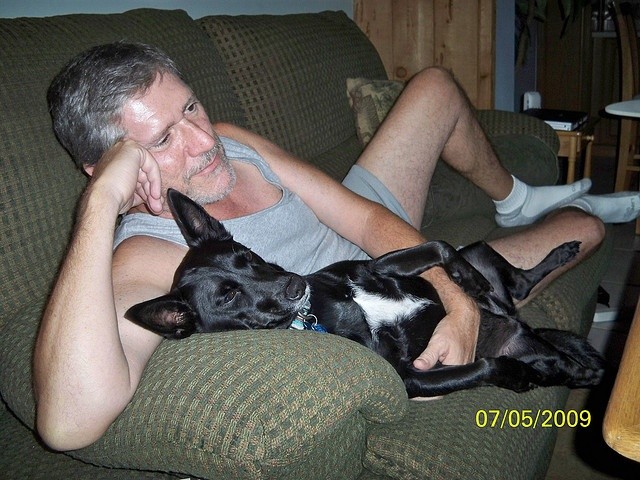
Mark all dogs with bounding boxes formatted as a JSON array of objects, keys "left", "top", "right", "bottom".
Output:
[{"left": 123, "top": 188, "right": 612, "bottom": 400}]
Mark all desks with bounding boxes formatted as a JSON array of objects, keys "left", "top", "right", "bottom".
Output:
[{"left": 555, "top": 117, "right": 600, "bottom": 185}]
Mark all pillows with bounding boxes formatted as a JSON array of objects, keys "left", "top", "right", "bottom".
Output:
[{"left": 346, "top": 76, "right": 498, "bottom": 230}]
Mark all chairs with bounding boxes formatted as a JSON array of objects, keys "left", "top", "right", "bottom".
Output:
[{"left": 599, "top": 1, "right": 638, "bottom": 226}]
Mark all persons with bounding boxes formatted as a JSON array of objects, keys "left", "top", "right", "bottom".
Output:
[{"left": 32, "top": 43, "right": 640, "bottom": 452}]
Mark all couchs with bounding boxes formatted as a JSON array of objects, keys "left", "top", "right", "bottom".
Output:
[{"left": 3, "top": 11, "right": 600, "bottom": 472}]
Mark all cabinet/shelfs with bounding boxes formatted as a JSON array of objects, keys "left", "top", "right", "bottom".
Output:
[
  {"left": 351, "top": 0, "right": 496, "bottom": 109},
  {"left": 512, "top": 1, "right": 638, "bottom": 158}
]
